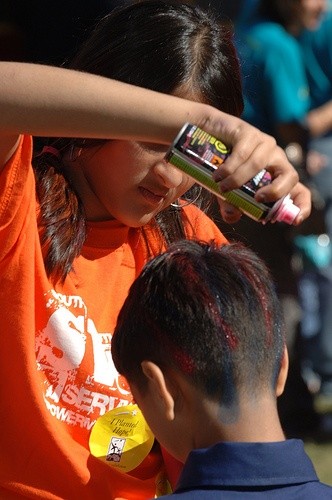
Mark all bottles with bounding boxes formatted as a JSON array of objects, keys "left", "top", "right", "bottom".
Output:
[{"left": 164, "top": 123, "right": 302, "bottom": 226}]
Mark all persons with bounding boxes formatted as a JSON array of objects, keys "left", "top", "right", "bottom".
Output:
[
  {"left": 230, "top": 0, "right": 332, "bottom": 446},
  {"left": 1, "top": 1, "right": 314, "bottom": 500},
  {"left": 106, "top": 233, "right": 332, "bottom": 500}
]
